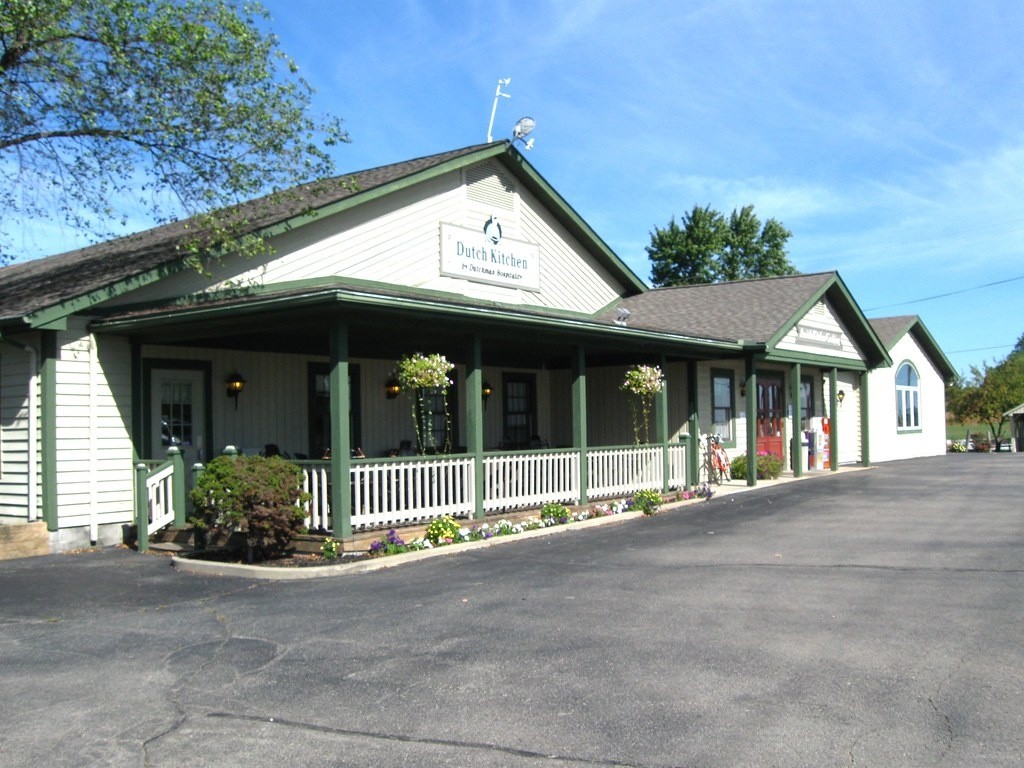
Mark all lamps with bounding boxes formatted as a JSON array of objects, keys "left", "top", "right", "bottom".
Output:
[
  {"left": 226, "top": 371, "right": 247, "bottom": 412},
  {"left": 482, "top": 381, "right": 494, "bottom": 410},
  {"left": 838, "top": 390, "right": 845, "bottom": 403},
  {"left": 385, "top": 371, "right": 402, "bottom": 401}
]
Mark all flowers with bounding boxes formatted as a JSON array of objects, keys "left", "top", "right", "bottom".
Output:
[
  {"left": 399, "top": 351, "right": 454, "bottom": 456},
  {"left": 617, "top": 363, "right": 666, "bottom": 445}
]
